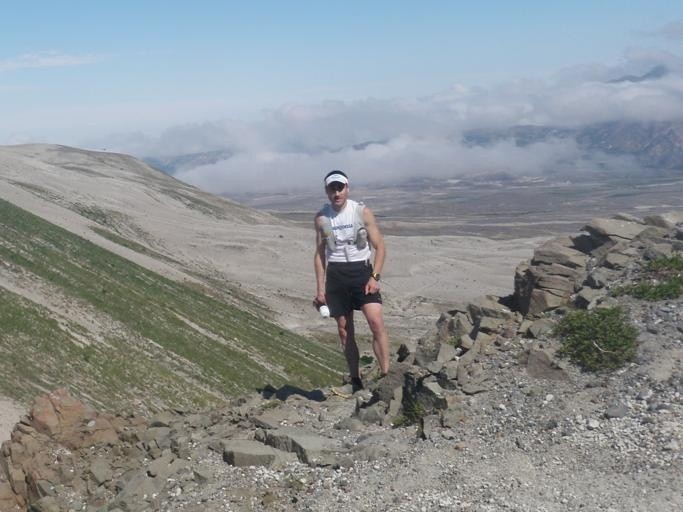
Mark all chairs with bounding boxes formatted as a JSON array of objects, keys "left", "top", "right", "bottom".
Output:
[{"left": 331, "top": 376, "right": 361, "bottom": 399}]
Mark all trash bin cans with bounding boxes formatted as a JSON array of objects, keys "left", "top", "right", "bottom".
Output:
[{"left": 370, "top": 272, "right": 381, "bottom": 281}]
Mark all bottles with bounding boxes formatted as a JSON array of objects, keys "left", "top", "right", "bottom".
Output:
[{"left": 325, "top": 174, "right": 348, "bottom": 187}]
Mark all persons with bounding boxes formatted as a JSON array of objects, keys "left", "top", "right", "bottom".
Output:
[{"left": 314, "top": 170, "right": 391, "bottom": 393}]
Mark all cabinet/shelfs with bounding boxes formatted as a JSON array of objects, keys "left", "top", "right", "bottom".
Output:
[{"left": 315, "top": 302, "right": 329, "bottom": 318}]
